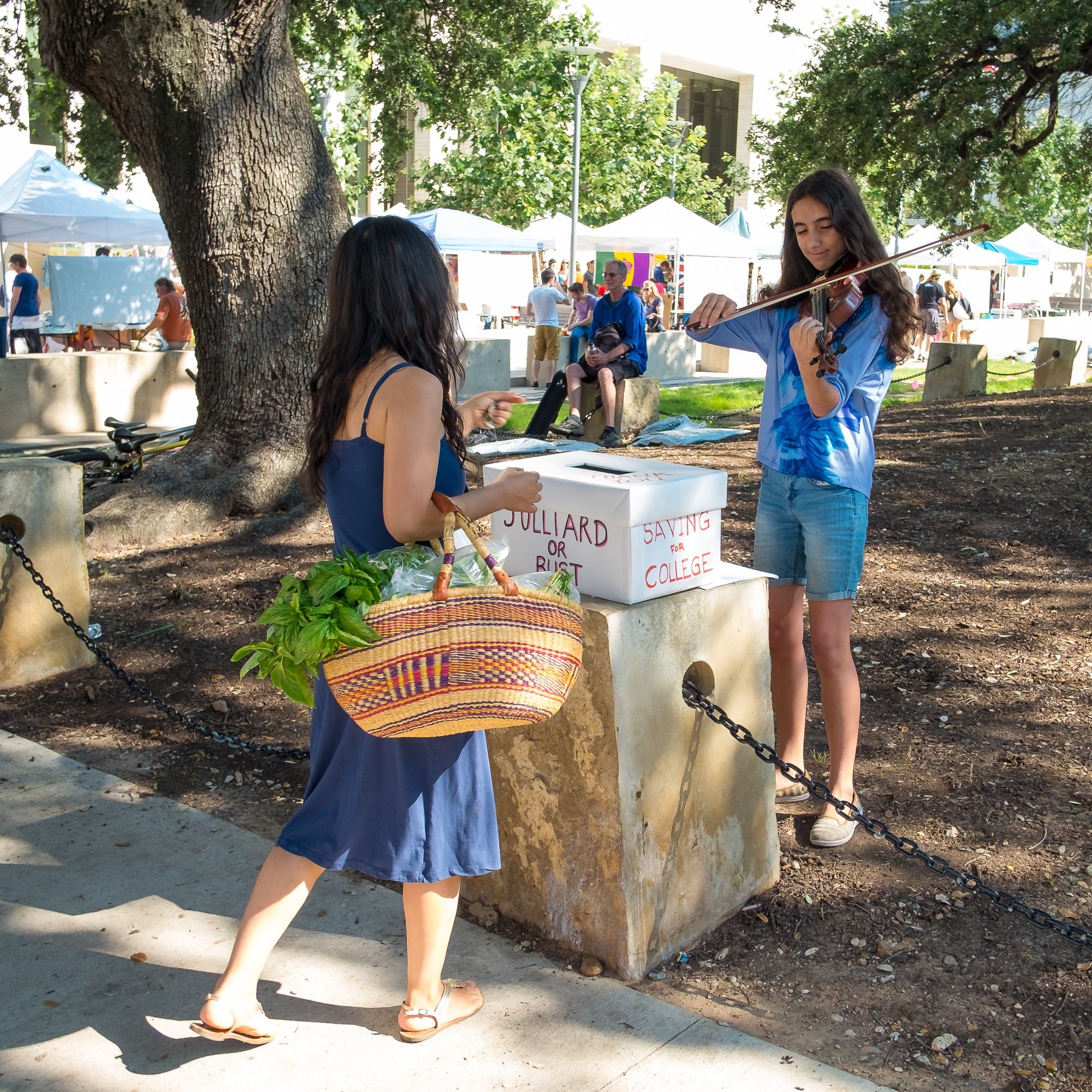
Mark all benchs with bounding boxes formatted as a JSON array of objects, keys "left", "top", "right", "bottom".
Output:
[
  {"left": 506, "top": 305, "right": 573, "bottom": 328},
  {"left": 1050, "top": 297, "right": 1092, "bottom": 316}
]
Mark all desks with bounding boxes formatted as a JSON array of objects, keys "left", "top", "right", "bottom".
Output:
[
  {"left": 39, "top": 327, "right": 79, "bottom": 352},
  {"left": 1011, "top": 308, "right": 1066, "bottom": 316},
  {"left": 476, "top": 314, "right": 520, "bottom": 328},
  {"left": 90, "top": 324, "right": 147, "bottom": 350}
]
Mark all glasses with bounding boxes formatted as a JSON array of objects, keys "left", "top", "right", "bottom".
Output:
[
  {"left": 602, "top": 273, "right": 619, "bottom": 278},
  {"left": 643, "top": 288, "right": 652, "bottom": 291},
  {"left": 563, "top": 262, "right": 568, "bottom": 264}
]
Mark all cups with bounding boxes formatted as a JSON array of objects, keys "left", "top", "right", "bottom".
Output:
[
  {"left": 130, "top": 340, "right": 140, "bottom": 351},
  {"left": 644, "top": 324, "right": 648, "bottom": 332},
  {"left": 561, "top": 328, "right": 568, "bottom": 335}
]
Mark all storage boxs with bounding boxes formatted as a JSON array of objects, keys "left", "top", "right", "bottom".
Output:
[{"left": 482, "top": 450, "right": 728, "bottom": 605}]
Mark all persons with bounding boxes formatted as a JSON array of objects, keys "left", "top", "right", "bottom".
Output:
[
  {"left": 189, "top": 217, "right": 545, "bottom": 1044},
  {"left": 682, "top": 166, "right": 930, "bottom": 844},
  {"left": 0, "top": 254, "right": 1000, "bottom": 446}
]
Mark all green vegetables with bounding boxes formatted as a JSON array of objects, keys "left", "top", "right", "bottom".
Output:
[{"left": 230, "top": 544, "right": 573, "bottom": 709}]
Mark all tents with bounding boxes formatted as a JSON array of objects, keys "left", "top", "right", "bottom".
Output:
[{"left": 0, "top": 150, "right": 1091, "bottom": 332}]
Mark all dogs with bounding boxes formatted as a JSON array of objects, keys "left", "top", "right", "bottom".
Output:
[{"left": 942, "top": 327, "right": 979, "bottom": 344}]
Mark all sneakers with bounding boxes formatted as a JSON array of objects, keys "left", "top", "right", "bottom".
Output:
[
  {"left": 550, "top": 416, "right": 585, "bottom": 437},
  {"left": 596, "top": 427, "right": 621, "bottom": 448}
]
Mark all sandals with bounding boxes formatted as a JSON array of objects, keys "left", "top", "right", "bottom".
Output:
[
  {"left": 188, "top": 990, "right": 273, "bottom": 1045},
  {"left": 400, "top": 978, "right": 485, "bottom": 1044}
]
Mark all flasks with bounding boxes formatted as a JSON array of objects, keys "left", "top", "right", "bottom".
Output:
[{"left": 587, "top": 340, "right": 599, "bottom": 368}]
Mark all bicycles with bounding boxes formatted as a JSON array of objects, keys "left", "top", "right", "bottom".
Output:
[{"left": 38, "top": 368, "right": 197, "bottom": 494}]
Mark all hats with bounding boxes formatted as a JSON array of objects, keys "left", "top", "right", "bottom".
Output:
[
  {"left": 931, "top": 270, "right": 942, "bottom": 276},
  {"left": 549, "top": 259, "right": 556, "bottom": 265}
]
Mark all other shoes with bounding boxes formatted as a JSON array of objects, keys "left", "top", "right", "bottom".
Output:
[
  {"left": 918, "top": 354, "right": 923, "bottom": 360},
  {"left": 531, "top": 383, "right": 539, "bottom": 390},
  {"left": 922, "top": 357, "right": 928, "bottom": 362},
  {"left": 810, "top": 791, "right": 864, "bottom": 848},
  {"left": 775, "top": 769, "right": 811, "bottom": 804},
  {"left": 545, "top": 383, "right": 549, "bottom": 389}
]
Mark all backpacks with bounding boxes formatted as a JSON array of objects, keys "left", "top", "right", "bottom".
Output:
[{"left": 582, "top": 323, "right": 623, "bottom": 375}]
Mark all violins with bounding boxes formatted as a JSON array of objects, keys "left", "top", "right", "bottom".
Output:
[{"left": 797, "top": 253, "right": 869, "bottom": 378}]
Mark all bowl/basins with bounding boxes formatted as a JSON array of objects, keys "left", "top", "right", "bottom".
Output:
[
  {"left": 126, "top": 248, "right": 140, "bottom": 257},
  {"left": 112, "top": 246, "right": 123, "bottom": 257}
]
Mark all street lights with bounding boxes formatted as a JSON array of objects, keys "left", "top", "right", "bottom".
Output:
[
  {"left": 558, "top": 44, "right": 600, "bottom": 304},
  {"left": 661, "top": 119, "right": 695, "bottom": 203}
]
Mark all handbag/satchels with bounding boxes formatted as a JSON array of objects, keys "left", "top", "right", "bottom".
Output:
[
  {"left": 646, "top": 318, "right": 662, "bottom": 332},
  {"left": 951, "top": 298, "right": 972, "bottom": 320},
  {"left": 322, "top": 490, "right": 586, "bottom": 740}
]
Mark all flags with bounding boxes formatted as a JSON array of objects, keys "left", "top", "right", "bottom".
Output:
[{"left": 593, "top": 252, "right": 650, "bottom": 287}]
